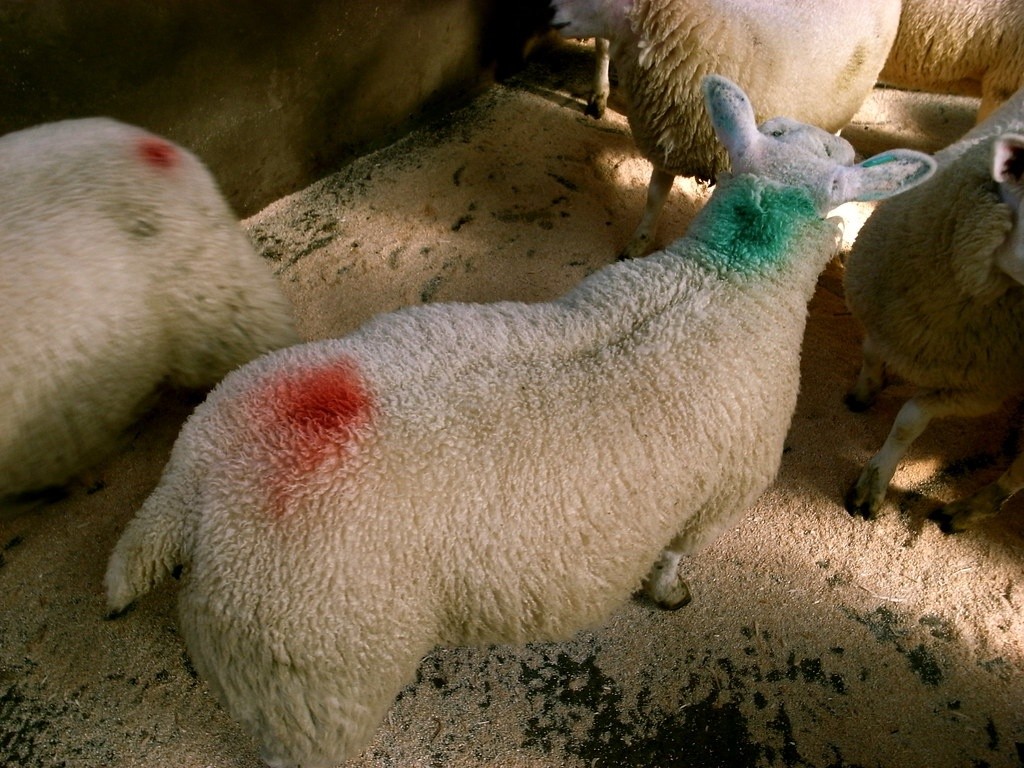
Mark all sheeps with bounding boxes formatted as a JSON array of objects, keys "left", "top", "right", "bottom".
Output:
[
  {"left": 0, "top": 118, "right": 298, "bottom": 498},
  {"left": 842, "top": 86, "right": 1024, "bottom": 533},
  {"left": 882, "top": 1, "right": 1023, "bottom": 128},
  {"left": 100, "top": 81, "right": 936, "bottom": 768},
  {"left": 551, "top": 0, "right": 903, "bottom": 263}
]
